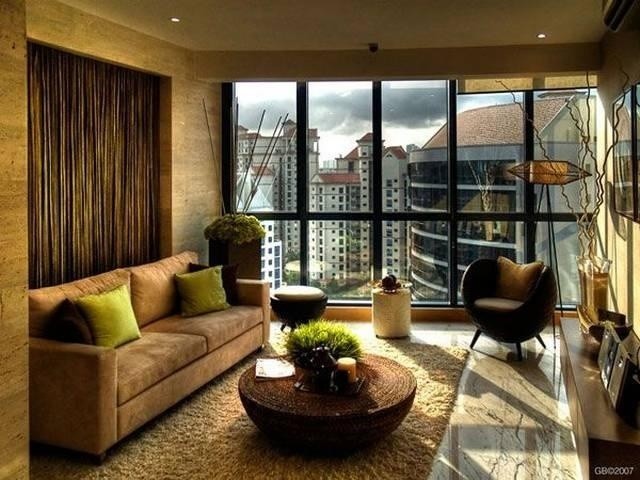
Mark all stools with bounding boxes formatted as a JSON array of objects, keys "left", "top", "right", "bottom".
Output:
[{"left": 269, "top": 283, "right": 329, "bottom": 330}]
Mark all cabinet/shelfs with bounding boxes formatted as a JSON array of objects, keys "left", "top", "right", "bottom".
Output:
[
  {"left": 203, "top": 213, "right": 266, "bottom": 282},
  {"left": 552, "top": 309, "right": 640, "bottom": 479}
]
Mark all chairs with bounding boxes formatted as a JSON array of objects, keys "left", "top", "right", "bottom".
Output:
[{"left": 455, "top": 249, "right": 555, "bottom": 359}]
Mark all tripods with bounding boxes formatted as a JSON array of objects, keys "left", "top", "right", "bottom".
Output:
[{"left": 529, "top": 185, "right": 564, "bottom": 348}]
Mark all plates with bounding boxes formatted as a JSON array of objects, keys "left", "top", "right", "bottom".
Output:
[
  {"left": 294, "top": 372, "right": 365, "bottom": 396},
  {"left": 366, "top": 278, "right": 413, "bottom": 291}
]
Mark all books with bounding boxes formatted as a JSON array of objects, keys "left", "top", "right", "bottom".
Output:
[{"left": 253, "top": 356, "right": 297, "bottom": 381}]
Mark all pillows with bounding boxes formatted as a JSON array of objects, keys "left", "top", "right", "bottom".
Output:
[
  {"left": 53, "top": 263, "right": 242, "bottom": 355},
  {"left": 496, "top": 253, "right": 545, "bottom": 303}
]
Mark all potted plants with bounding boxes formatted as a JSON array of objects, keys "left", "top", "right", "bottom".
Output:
[{"left": 276, "top": 321, "right": 363, "bottom": 395}]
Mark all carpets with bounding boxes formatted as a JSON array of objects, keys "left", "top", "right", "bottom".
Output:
[{"left": 27, "top": 336, "right": 473, "bottom": 480}]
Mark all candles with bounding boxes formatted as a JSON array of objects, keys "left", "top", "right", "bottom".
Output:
[{"left": 335, "top": 356, "right": 357, "bottom": 385}]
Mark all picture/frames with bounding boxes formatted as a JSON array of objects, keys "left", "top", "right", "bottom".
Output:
[
  {"left": 635, "top": 80, "right": 640, "bottom": 226},
  {"left": 610, "top": 83, "right": 635, "bottom": 221}
]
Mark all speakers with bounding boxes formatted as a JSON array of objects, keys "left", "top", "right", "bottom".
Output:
[
  {"left": 596, "top": 323, "right": 633, "bottom": 372},
  {"left": 608, "top": 344, "right": 640, "bottom": 410}
]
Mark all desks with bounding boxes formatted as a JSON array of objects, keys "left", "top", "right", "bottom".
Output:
[{"left": 365, "top": 275, "right": 417, "bottom": 342}]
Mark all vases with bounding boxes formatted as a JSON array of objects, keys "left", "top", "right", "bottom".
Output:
[{"left": 576, "top": 255, "right": 612, "bottom": 323}]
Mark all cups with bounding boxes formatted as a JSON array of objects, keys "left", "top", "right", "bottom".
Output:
[{"left": 337, "top": 357, "right": 357, "bottom": 384}]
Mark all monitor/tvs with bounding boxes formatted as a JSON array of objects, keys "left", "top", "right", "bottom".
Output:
[{"left": 611, "top": 81, "right": 640, "bottom": 223}]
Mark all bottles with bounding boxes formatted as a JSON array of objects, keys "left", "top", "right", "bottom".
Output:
[{"left": 311, "top": 343, "right": 337, "bottom": 392}]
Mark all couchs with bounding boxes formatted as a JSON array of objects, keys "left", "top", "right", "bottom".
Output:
[{"left": 25, "top": 248, "right": 275, "bottom": 468}]
